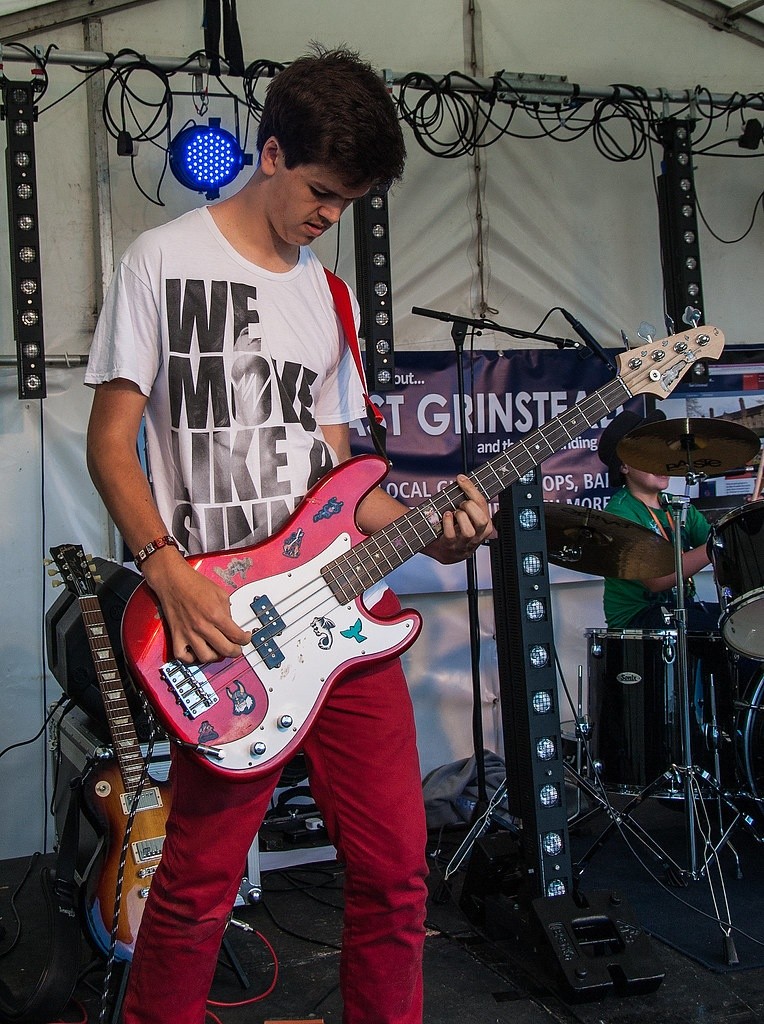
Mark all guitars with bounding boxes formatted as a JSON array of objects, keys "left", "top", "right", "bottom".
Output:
[
  {"left": 116, "top": 303, "right": 728, "bottom": 784},
  {"left": 45, "top": 539, "right": 172, "bottom": 962}
]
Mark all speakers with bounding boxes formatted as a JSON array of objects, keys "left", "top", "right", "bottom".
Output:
[{"left": 46, "top": 556, "right": 167, "bottom": 742}]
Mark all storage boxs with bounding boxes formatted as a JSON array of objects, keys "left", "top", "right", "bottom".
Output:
[{"left": 44, "top": 702, "right": 263, "bottom": 919}]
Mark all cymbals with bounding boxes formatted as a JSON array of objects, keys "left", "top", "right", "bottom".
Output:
[
  {"left": 543, "top": 501, "right": 684, "bottom": 582},
  {"left": 613, "top": 416, "right": 760, "bottom": 478}
]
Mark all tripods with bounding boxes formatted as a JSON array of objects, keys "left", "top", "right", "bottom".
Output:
[{"left": 406, "top": 303, "right": 764, "bottom": 965}]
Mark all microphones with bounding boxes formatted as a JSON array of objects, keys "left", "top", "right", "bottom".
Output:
[{"left": 560, "top": 308, "right": 616, "bottom": 376}]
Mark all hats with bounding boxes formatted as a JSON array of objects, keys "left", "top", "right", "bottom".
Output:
[{"left": 597, "top": 409, "right": 667, "bottom": 469}]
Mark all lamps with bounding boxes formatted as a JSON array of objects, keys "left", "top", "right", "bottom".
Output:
[{"left": 165, "top": 54, "right": 254, "bottom": 202}]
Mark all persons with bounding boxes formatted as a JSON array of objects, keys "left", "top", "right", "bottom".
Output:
[
  {"left": 595, "top": 408, "right": 716, "bottom": 632},
  {"left": 86, "top": 50, "right": 495, "bottom": 1024}
]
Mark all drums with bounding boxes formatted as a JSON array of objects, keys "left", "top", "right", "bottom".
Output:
[
  {"left": 704, "top": 495, "right": 764, "bottom": 663},
  {"left": 580, "top": 624, "right": 730, "bottom": 805},
  {"left": 691, "top": 643, "right": 764, "bottom": 801}
]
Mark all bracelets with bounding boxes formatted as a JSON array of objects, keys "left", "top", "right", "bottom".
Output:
[{"left": 132, "top": 536, "right": 180, "bottom": 571}]
